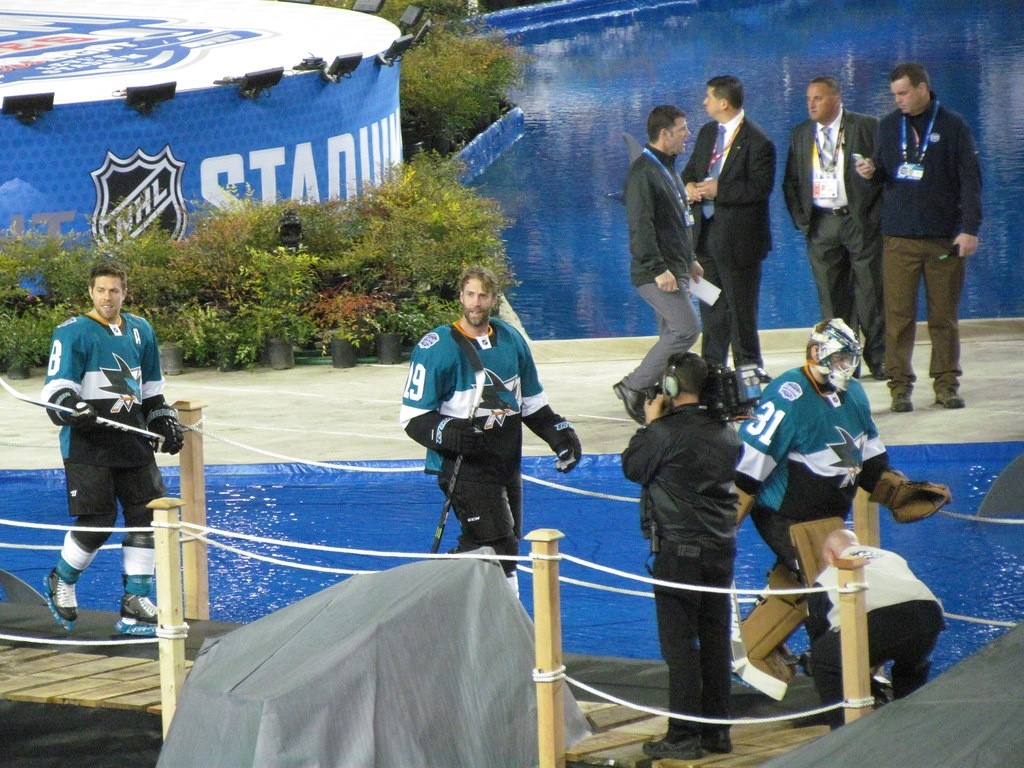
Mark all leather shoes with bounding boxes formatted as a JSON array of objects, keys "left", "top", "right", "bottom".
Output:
[{"left": 613, "top": 381, "right": 647, "bottom": 425}]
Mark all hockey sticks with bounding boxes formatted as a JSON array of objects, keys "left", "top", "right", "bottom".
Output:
[
  {"left": 429, "top": 328, "right": 488, "bottom": 560},
  {"left": 0, "top": 377, "right": 187, "bottom": 448},
  {"left": 728, "top": 577, "right": 789, "bottom": 703}
]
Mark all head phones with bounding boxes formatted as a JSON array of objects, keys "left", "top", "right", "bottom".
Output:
[{"left": 662, "top": 351, "right": 694, "bottom": 398}]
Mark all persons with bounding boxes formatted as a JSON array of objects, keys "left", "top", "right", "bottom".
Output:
[
  {"left": 782, "top": 76, "right": 889, "bottom": 379},
  {"left": 805, "top": 528, "right": 947, "bottom": 731},
  {"left": 43, "top": 265, "right": 185, "bottom": 635},
  {"left": 612, "top": 105, "right": 703, "bottom": 426},
  {"left": 855, "top": 63, "right": 984, "bottom": 412},
  {"left": 737, "top": 318, "right": 952, "bottom": 679},
  {"left": 400, "top": 267, "right": 582, "bottom": 599},
  {"left": 621, "top": 351, "right": 745, "bottom": 760},
  {"left": 681, "top": 74, "right": 776, "bottom": 369}
]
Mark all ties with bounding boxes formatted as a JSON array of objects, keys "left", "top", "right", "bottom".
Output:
[
  {"left": 820, "top": 126, "right": 836, "bottom": 179},
  {"left": 702, "top": 125, "right": 726, "bottom": 220}
]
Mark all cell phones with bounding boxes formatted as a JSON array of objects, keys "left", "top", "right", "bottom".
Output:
[{"left": 853, "top": 154, "right": 869, "bottom": 166}]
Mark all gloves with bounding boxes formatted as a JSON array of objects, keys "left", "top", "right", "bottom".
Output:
[
  {"left": 543, "top": 418, "right": 581, "bottom": 473},
  {"left": 54, "top": 392, "right": 97, "bottom": 430},
  {"left": 431, "top": 417, "right": 484, "bottom": 457},
  {"left": 145, "top": 405, "right": 184, "bottom": 455}
]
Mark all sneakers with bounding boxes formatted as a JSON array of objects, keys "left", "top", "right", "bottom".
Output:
[
  {"left": 891, "top": 395, "right": 913, "bottom": 412},
  {"left": 643, "top": 738, "right": 704, "bottom": 760},
  {"left": 116, "top": 574, "right": 160, "bottom": 637},
  {"left": 43, "top": 567, "right": 78, "bottom": 632},
  {"left": 935, "top": 390, "right": 965, "bottom": 409},
  {"left": 870, "top": 363, "right": 889, "bottom": 380},
  {"left": 700, "top": 731, "right": 733, "bottom": 753}
]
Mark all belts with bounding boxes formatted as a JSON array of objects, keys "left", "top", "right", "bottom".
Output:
[{"left": 813, "top": 206, "right": 849, "bottom": 216}]
